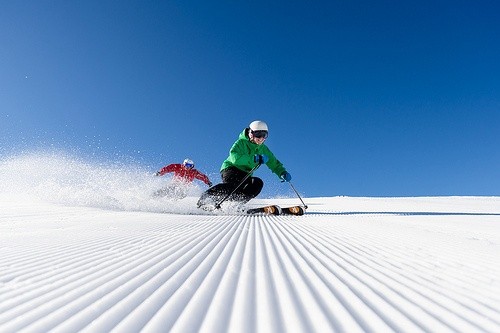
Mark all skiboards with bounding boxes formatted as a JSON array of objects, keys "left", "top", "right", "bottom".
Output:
[{"left": 241, "top": 204, "right": 304, "bottom": 216}]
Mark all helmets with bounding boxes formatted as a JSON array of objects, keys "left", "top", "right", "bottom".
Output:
[
  {"left": 250, "top": 121, "right": 268, "bottom": 132},
  {"left": 184, "top": 159, "right": 193, "bottom": 166}
]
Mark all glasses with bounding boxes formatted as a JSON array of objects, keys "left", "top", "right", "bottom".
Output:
[
  {"left": 251, "top": 131, "right": 268, "bottom": 139},
  {"left": 186, "top": 163, "right": 194, "bottom": 168}
]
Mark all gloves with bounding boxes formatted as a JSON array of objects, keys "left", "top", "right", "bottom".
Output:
[
  {"left": 208, "top": 182, "right": 212, "bottom": 187},
  {"left": 280, "top": 172, "right": 291, "bottom": 182},
  {"left": 254, "top": 154, "right": 269, "bottom": 164},
  {"left": 155, "top": 171, "right": 160, "bottom": 176}
]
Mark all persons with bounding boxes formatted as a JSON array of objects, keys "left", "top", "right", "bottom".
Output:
[
  {"left": 197, "top": 120, "right": 292, "bottom": 211},
  {"left": 152, "top": 158, "right": 212, "bottom": 198}
]
